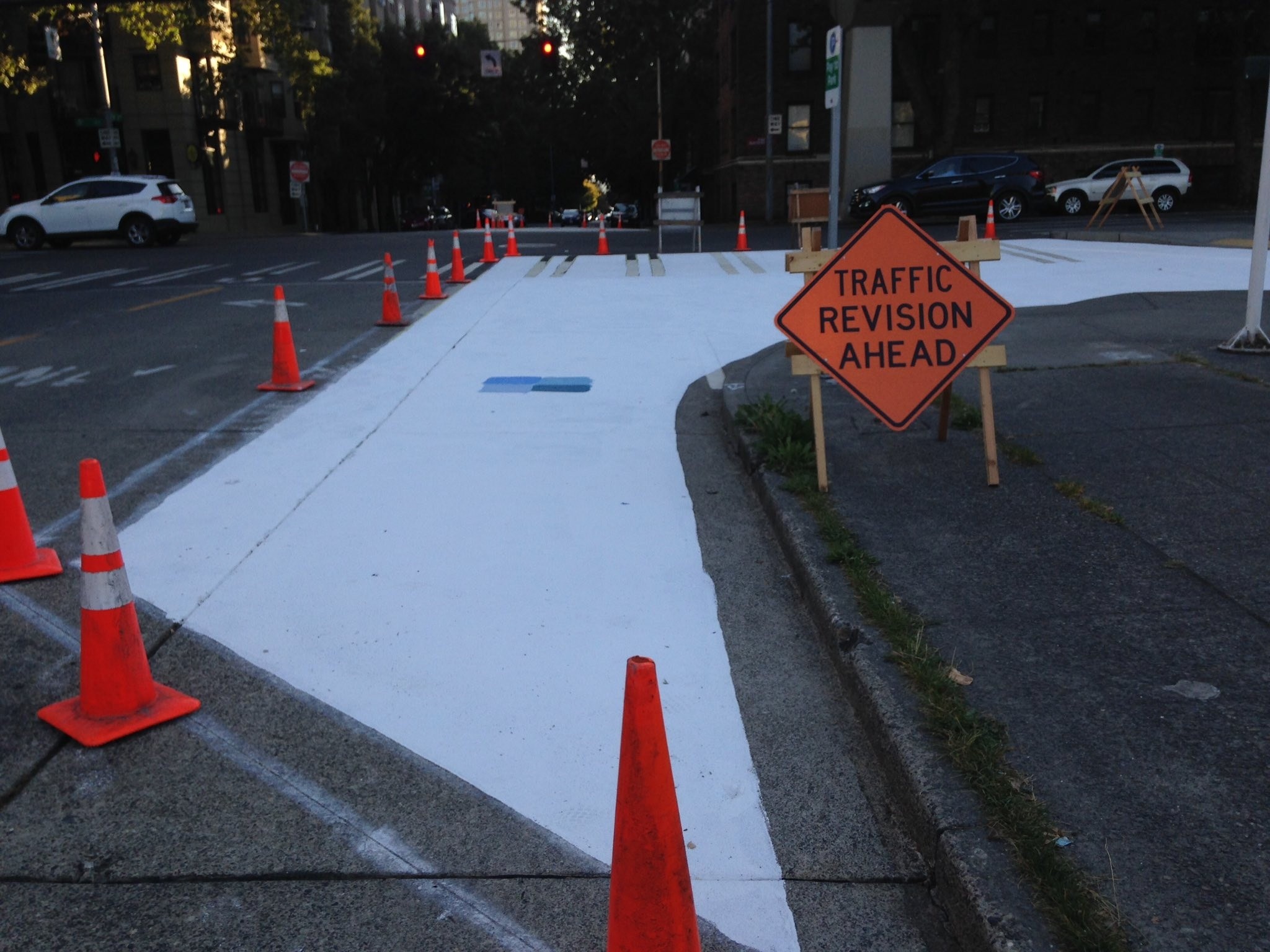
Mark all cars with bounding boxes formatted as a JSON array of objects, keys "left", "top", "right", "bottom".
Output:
[
  {"left": 403, "top": 206, "right": 456, "bottom": 231},
  {"left": 558, "top": 209, "right": 583, "bottom": 227}
]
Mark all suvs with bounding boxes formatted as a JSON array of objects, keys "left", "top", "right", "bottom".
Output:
[
  {"left": 611, "top": 202, "right": 641, "bottom": 228},
  {"left": 847, "top": 153, "right": 1049, "bottom": 224},
  {"left": 1045, "top": 157, "right": 1194, "bottom": 217},
  {"left": 0, "top": 173, "right": 196, "bottom": 253}
]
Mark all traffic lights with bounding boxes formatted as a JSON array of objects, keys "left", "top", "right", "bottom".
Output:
[
  {"left": 84, "top": 147, "right": 109, "bottom": 170},
  {"left": 541, "top": 38, "right": 557, "bottom": 69},
  {"left": 415, "top": 44, "right": 427, "bottom": 70}
]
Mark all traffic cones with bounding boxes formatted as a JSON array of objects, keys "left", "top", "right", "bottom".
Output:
[
  {"left": 445, "top": 230, "right": 471, "bottom": 284},
  {"left": 582, "top": 213, "right": 587, "bottom": 228},
  {"left": 984, "top": 199, "right": 1000, "bottom": 240},
  {"left": 464, "top": 207, "right": 528, "bottom": 263},
  {"left": 255, "top": 284, "right": 316, "bottom": 391},
  {"left": 548, "top": 213, "right": 552, "bottom": 228},
  {"left": 0, "top": 429, "right": 63, "bottom": 585},
  {"left": 732, "top": 210, "right": 752, "bottom": 251},
  {"left": 36, "top": 457, "right": 201, "bottom": 749},
  {"left": 595, "top": 213, "right": 611, "bottom": 256},
  {"left": 616, "top": 214, "right": 624, "bottom": 229},
  {"left": 418, "top": 239, "right": 449, "bottom": 299},
  {"left": 373, "top": 252, "right": 410, "bottom": 327},
  {"left": 606, "top": 654, "right": 703, "bottom": 952}
]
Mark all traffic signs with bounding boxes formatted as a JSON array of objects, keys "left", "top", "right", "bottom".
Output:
[
  {"left": 768, "top": 114, "right": 782, "bottom": 135},
  {"left": 481, "top": 50, "right": 503, "bottom": 78}
]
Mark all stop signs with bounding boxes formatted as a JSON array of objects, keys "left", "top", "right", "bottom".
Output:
[
  {"left": 290, "top": 160, "right": 309, "bottom": 182},
  {"left": 651, "top": 139, "right": 672, "bottom": 161}
]
